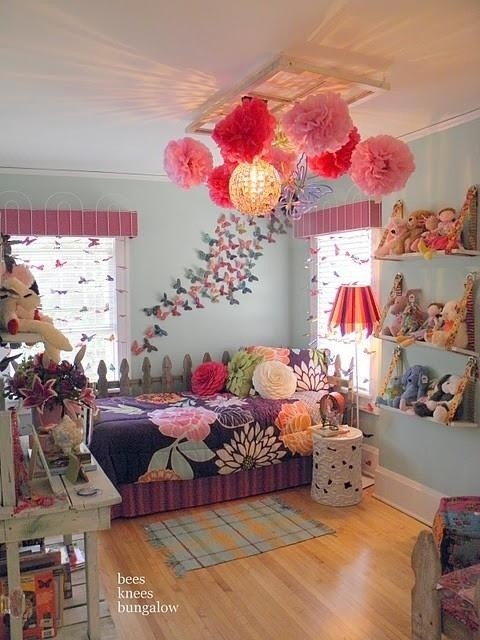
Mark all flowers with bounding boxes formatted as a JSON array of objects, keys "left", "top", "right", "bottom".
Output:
[{"left": 1, "top": 345, "right": 98, "bottom": 420}]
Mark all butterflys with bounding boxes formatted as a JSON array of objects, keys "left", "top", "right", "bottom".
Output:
[
  {"left": 130, "top": 211, "right": 293, "bottom": 356},
  {"left": 6, "top": 236, "right": 117, "bottom": 372},
  {"left": 38, "top": 579, "right": 52, "bottom": 588},
  {"left": 304, "top": 229, "right": 375, "bottom": 386}
]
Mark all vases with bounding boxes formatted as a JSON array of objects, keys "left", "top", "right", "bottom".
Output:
[{"left": 31, "top": 405, "right": 62, "bottom": 452}]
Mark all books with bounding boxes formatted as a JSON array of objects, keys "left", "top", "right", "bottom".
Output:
[{"left": 1, "top": 538, "right": 74, "bottom": 639}]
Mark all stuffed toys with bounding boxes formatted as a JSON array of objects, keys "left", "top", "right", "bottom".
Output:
[
  {"left": 0, "top": 260, "right": 73, "bottom": 369},
  {"left": 373, "top": 205, "right": 468, "bottom": 422}
]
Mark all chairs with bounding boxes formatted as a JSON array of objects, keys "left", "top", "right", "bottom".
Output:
[
  {"left": 432, "top": 496, "right": 480, "bottom": 575},
  {"left": 411, "top": 529, "right": 480, "bottom": 640}
]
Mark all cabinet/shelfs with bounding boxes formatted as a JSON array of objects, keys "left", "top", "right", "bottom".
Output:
[
  {"left": 371, "top": 249, "right": 480, "bottom": 428},
  {"left": 1, "top": 329, "right": 122, "bottom": 640}
]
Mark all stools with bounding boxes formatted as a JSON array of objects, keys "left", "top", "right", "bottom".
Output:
[{"left": 310, "top": 427, "right": 364, "bottom": 507}]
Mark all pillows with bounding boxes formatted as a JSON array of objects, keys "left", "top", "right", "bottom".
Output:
[{"left": 191, "top": 349, "right": 330, "bottom": 400}]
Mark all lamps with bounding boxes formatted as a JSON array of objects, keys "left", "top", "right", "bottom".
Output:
[
  {"left": 326, "top": 281, "right": 380, "bottom": 489},
  {"left": 226, "top": 95, "right": 282, "bottom": 216}
]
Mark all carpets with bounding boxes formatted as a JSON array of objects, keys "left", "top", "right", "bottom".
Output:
[{"left": 141, "top": 494, "right": 336, "bottom": 578}]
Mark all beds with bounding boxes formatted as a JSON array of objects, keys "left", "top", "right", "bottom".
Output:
[{"left": 90, "top": 354, "right": 346, "bottom": 519}]
